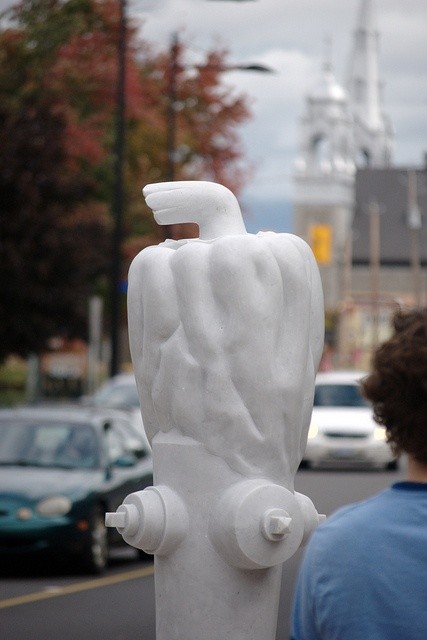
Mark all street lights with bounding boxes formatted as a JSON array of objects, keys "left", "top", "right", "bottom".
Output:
[{"left": 152, "top": 47, "right": 277, "bottom": 245}]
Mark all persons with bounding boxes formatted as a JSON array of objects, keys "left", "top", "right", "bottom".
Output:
[{"left": 289, "top": 304, "right": 427, "bottom": 639}]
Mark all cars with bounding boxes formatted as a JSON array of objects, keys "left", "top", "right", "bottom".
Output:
[
  {"left": 0, "top": 406, "right": 159, "bottom": 573},
  {"left": 299, "top": 371, "right": 401, "bottom": 473},
  {"left": 78, "top": 373, "right": 163, "bottom": 462}
]
